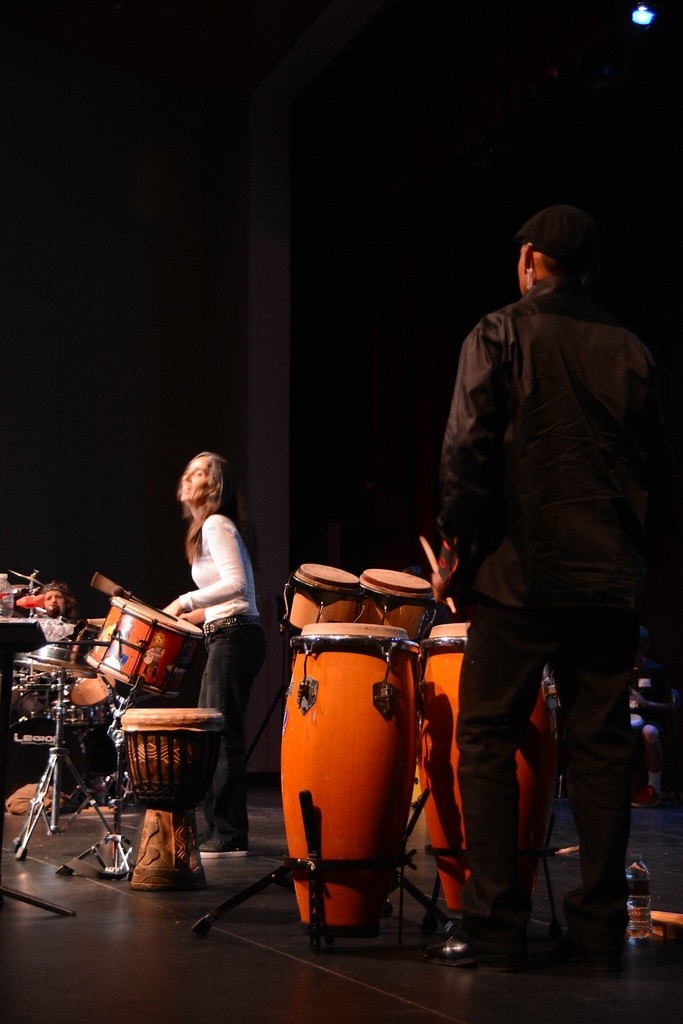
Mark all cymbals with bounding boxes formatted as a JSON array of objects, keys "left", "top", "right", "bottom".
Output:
[
  {"left": 8, "top": 568, "right": 46, "bottom": 588},
  {"left": 13, "top": 654, "right": 100, "bottom": 678}
]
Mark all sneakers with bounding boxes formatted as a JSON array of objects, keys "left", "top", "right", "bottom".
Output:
[
  {"left": 195, "top": 832, "right": 209, "bottom": 845},
  {"left": 200, "top": 838, "right": 250, "bottom": 858}
]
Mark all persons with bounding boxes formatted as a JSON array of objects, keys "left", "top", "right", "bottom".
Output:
[
  {"left": 158, "top": 451, "right": 269, "bottom": 857},
  {"left": 419, "top": 204, "right": 674, "bottom": 974},
  {"left": 628, "top": 624, "right": 673, "bottom": 808},
  {"left": 12, "top": 581, "right": 84, "bottom": 649}
]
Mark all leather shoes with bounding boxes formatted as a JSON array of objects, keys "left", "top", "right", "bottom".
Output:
[
  {"left": 561, "top": 936, "right": 614, "bottom": 970},
  {"left": 417, "top": 933, "right": 525, "bottom": 970}
]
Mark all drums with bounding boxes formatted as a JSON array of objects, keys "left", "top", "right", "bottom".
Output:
[
  {"left": 631, "top": 712, "right": 644, "bottom": 794},
  {"left": 287, "top": 562, "right": 362, "bottom": 631},
  {"left": 418, "top": 622, "right": 558, "bottom": 916},
  {"left": 68, "top": 674, "right": 117, "bottom": 708},
  {"left": 120, "top": 707, "right": 225, "bottom": 892},
  {"left": 280, "top": 622, "right": 418, "bottom": 938},
  {"left": 357, "top": 568, "right": 433, "bottom": 641},
  {"left": 84, "top": 594, "right": 204, "bottom": 698},
  {"left": 5, "top": 718, "right": 85, "bottom": 798},
  {"left": 49, "top": 700, "right": 114, "bottom": 726}
]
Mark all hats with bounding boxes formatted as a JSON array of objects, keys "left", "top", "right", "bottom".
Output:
[{"left": 514, "top": 205, "right": 593, "bottom": 259}]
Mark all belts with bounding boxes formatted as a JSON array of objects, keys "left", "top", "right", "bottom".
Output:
[{"left": 203, "top": 615, "right": 263, "bottom": 636}]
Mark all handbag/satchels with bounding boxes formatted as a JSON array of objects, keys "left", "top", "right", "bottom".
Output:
[{"left": 5, "top": 783, "right": 66, "bottom": 815}]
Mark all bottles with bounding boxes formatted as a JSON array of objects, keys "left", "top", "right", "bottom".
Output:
[
  {"left": 626, "top": 854, "right": 652, "bottom": 937},
  {"left": 97, "top": 776, "right": 106, "bottom": 804},
  {"left": 0, "top": 573, "right": 14, "bottom": 617}
]
